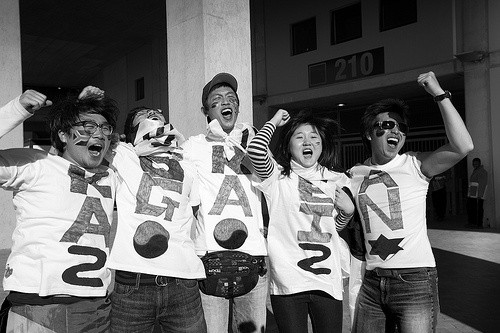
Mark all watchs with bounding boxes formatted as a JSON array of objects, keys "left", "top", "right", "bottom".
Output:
[{"left": 434, "top": 91, "right": 451, "bottom": 102}]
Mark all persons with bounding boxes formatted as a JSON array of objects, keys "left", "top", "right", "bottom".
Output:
[
  {"left": 247, "top": 108, "right": 356, "bottom": 333},
  {"left": 345, "top": 71, "right": 475, "bottom": 333},
  {"left": 77, "top": 85, "right": 209, "bottom": 333},
  {"left": 178, "top": 73, "right": 270, "bottom": 333},
  {"left": 430, "top": 171, "right": 446, "bottom": 221},
  {"left": 0, "top": 89, "right": 117, "bottom": 333},
  {"left": 464, "top": 157, "right": 488, "bottom": 229}
]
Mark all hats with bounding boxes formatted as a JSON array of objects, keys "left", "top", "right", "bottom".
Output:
[
  {"left": 202, "top": 73, "right": 237, "bottom": 103},
  {"left": 124, "top": 106, "right": 149, "bottom": 136}
]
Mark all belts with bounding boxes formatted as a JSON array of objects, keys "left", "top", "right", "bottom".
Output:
[
  {"left": 115, "top": 272, "right": 174, "bottom": 287},
  {"left": 365, "top": 267, "right": 436, "bottom": 277}
]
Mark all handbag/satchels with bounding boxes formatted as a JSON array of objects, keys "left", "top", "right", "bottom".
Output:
[{"left": 199, "top": 250, "right": 268, "bottom": 298}]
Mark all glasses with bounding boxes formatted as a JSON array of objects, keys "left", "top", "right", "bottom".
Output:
[
  {"left": 72, "top": 120, "right": 113, "bottom": 136},
  {"left": 372, "top": 121, "right": 408, "bottom": 133}
]
